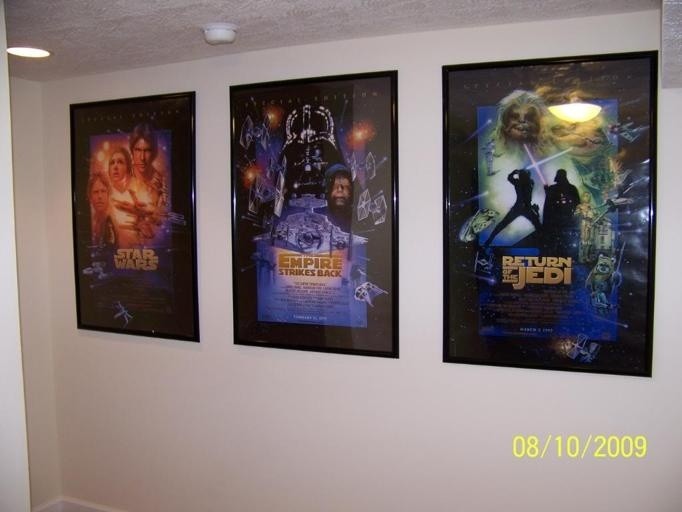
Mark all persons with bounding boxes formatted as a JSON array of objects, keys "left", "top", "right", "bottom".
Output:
[
  {"left": 540, "top": 168, "right": 582, "bottom": 252},
  {"left": 323, "top": 166, "right": 352, "bottom": 230},
  {"left": 126, "top": 116, "right": 169, "bottom": 202},
  {"left": 481, "top": 166, "right": 543, "bottom": 253},
  {"left": 492, "top": 90, "right": 557, "bottom": 143},
  {"left": 103, "top": 147, "right": 161, "bottom": 236},
  {"left": 88, "top": 172, "right": 118, "bottom": 247}
]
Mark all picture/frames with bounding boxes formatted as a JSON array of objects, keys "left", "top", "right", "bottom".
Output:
[
  {"left": 442, "top": 49, "right": 658, "bottom": 378},
  {"left": 229, "top": 69, "right": 399, "bottom": 359},
  {"left": 69, "top": 91, "right": 201, "bottom": 342}
]
